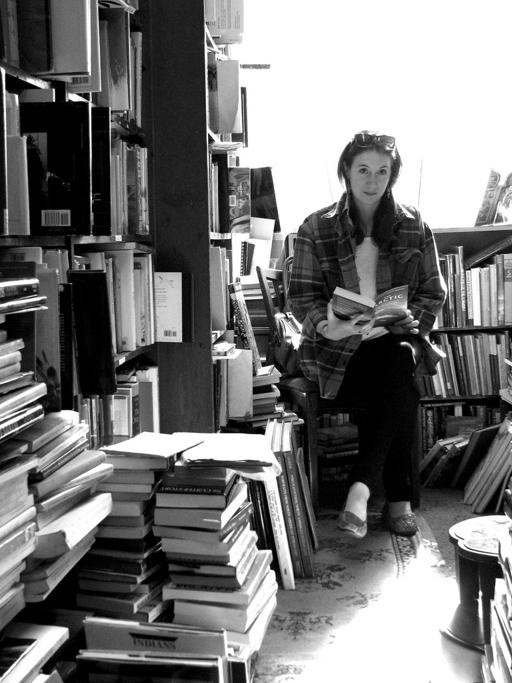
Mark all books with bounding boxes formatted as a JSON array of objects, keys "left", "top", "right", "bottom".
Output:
[
  {"left": 417, "top": 168, "right": 512, "bottom": 682},
  {"left": 2, "top": 0, "right": 317, "bottom": 683},
  {"left": 332, "top": 283, "right": 409, "bottom": 328}
]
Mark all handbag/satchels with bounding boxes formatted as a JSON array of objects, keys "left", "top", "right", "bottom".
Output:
[{"left": 272, "top": 312, "right": 303, "bottom": 374}]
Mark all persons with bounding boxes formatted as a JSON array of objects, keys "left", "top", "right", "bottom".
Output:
[{"left": 285, "top": 128, "right": 448, "bottom": 539}]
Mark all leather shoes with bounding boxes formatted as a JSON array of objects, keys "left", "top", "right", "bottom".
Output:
[
  {"left": 381, "top": 503, "right": 418, "bottom": 536},
  {"left": 336, "top": 503, "right": 368, "bottom": 540}
]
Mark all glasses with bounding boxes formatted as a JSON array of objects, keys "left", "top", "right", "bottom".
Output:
[{"left": 346, "top": 134, "right": 398, "bottom": 156}]
